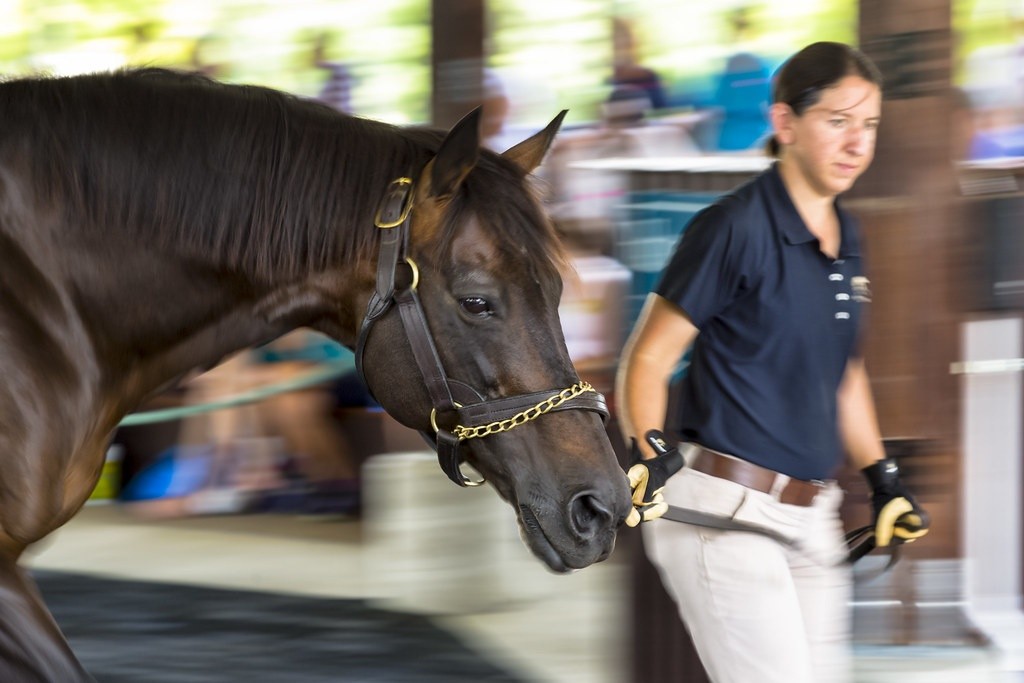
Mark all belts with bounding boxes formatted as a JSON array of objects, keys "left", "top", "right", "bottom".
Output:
[{"left": 676, "top": 441, "right": 824, "bottom": 507}]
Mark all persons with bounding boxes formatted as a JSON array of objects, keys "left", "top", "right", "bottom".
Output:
[{"left": 613, "top": 40, "right": 936, "bottom": 682}]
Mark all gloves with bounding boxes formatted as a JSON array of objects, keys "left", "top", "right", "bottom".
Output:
[
  {"left": 859, "top": 457, "right": 928, "bottom": 547},
  {"left": 626, "top": 458, "right": 668, "bottom": 527}
]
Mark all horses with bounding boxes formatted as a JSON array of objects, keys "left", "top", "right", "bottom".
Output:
[{"left": 1, "top": 64, "right": 634, "bottom": 683}]
